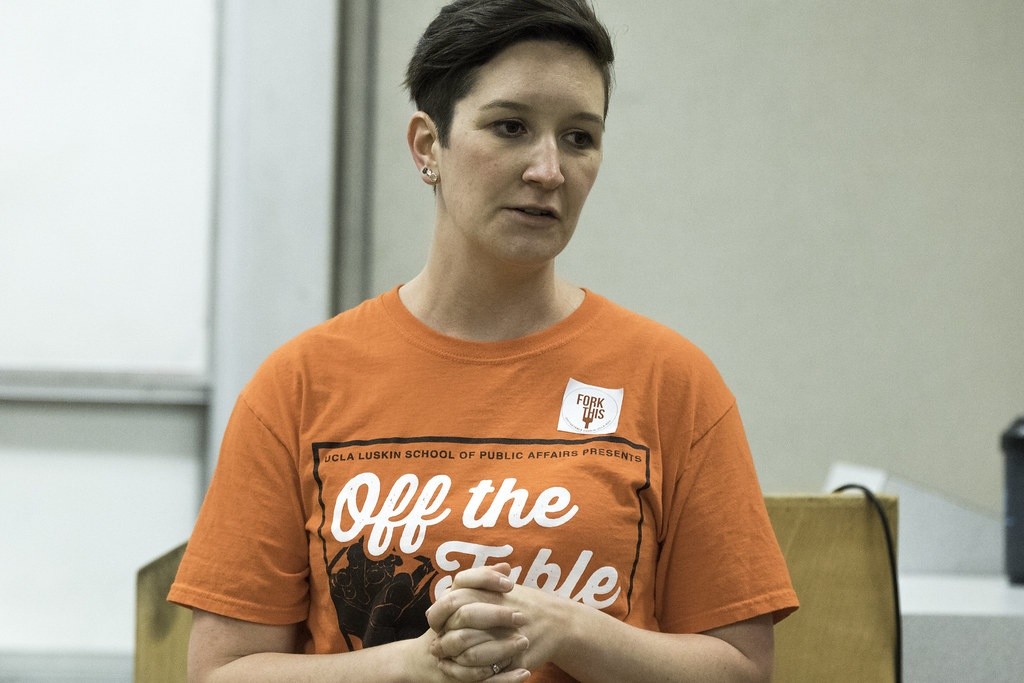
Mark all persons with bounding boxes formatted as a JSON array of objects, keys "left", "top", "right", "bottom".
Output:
[{"left": 167, "top": 0, "right": 801, "bottom": 683}]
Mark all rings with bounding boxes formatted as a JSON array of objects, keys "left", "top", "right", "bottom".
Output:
[{"left": 491, "top": 663, "right": 502, "bottom": 674}]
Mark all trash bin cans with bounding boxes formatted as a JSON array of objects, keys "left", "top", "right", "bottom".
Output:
[{"left": 999, "top": 415, "right": 1024, "bottom": 585}]
